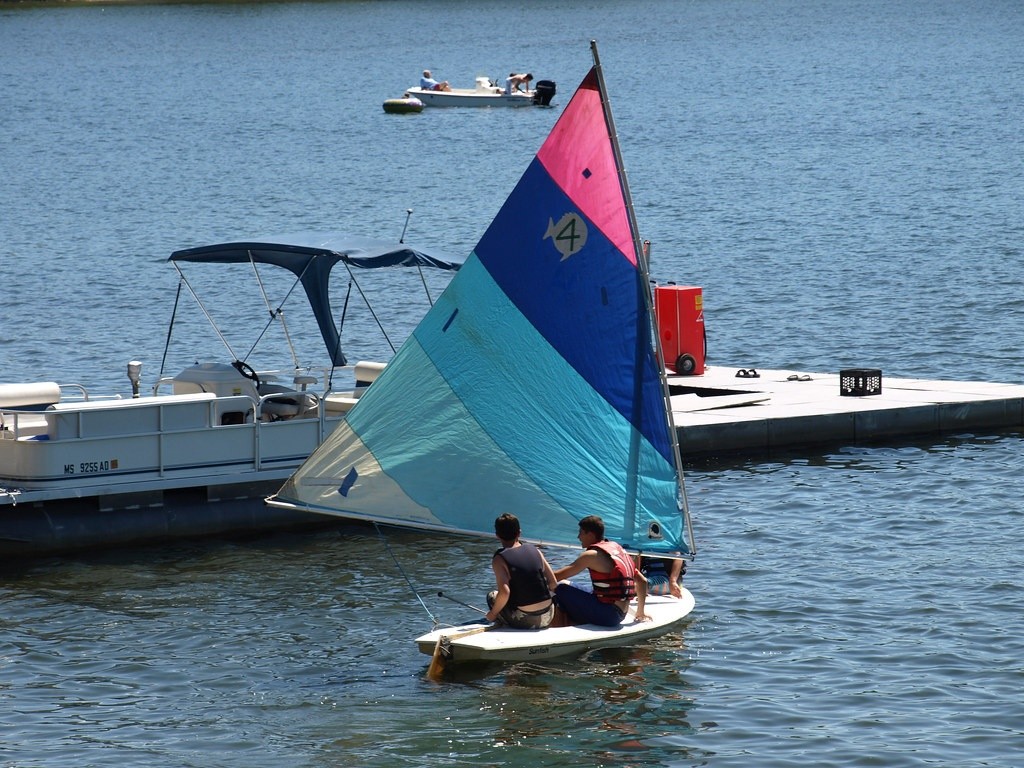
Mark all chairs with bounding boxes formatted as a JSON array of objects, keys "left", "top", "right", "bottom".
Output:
[{"left": 475, "top": 77, "right": 498, "bottom": 94}]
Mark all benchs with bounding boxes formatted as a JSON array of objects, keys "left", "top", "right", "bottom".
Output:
[{"left": 0, "top": 382, "right": 63, "bottom": 439}]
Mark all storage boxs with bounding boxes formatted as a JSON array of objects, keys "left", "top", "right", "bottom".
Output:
[{"left": 840, "top": 368, "right": 882, "bottom": 397}]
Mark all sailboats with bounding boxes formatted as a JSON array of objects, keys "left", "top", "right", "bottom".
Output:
[{"left": 262, "top": 36, "right": 698, "bottom": 668}]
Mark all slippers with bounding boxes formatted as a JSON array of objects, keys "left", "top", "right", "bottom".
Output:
[
  {"left": 787, "top": 374, "right": 798, "bottom": 380},
  {"left": 735, "top": 370, "right": 750, "bottom": 378},
  {"left": 747, "top": 370, "right": 760, "bottom": 378},
  {"left": 798, "top": 375, "right": 810, "bottom": 381}
]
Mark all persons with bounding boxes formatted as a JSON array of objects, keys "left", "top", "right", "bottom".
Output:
[
  {"left": 636, "top": 556, "right": 686, "bottom": 599},
  {"left": 484, "top": 513, "right": 558, "bottom": 629},
  {"left": 554, "top": 515, "right": 653, "bottom": 626},
  {"left": 420, "top": 70, "right": 451, "bottom": 92},
  {"left": 496, "top": 72, "right": 534, "bottom": 94}
]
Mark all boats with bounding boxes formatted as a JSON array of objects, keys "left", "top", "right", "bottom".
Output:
[
  {"left": 0, "top": 241, "right": 464, "bottom": 544},
  {"left": 408, "top": 80, "right": 557, "bottom": 107}
]
